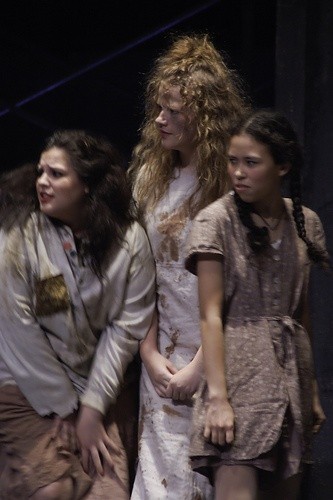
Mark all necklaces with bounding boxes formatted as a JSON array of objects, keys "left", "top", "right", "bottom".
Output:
[{"left": 251, "top": 204, "right": 286, "bottom": 229}]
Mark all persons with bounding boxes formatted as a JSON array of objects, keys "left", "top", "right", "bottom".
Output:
[
  {"left": 0, "top": 130, "right": 157, "bottom": 500},
  {"left": 124, "top": 34, "right": 259, "bottom": 500},
  {"left": 182, "top": 113, "right": 333, "bottom": 500}
]
